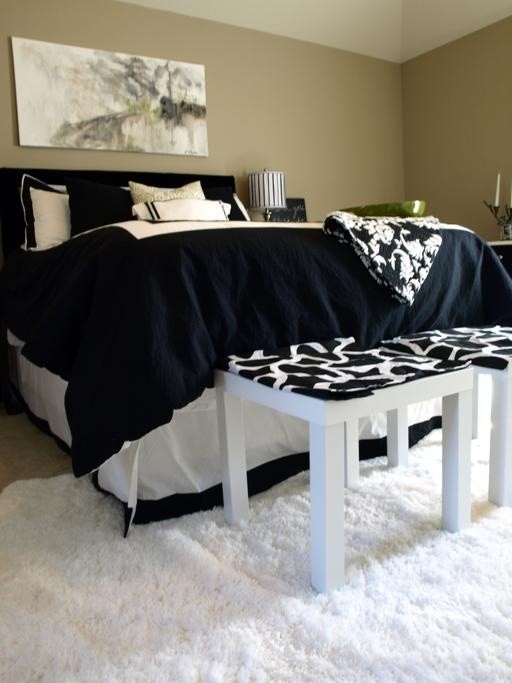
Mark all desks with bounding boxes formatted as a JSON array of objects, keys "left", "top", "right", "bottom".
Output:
[{"left": 486, "top": 241, "right": 512, "bottom": 280}]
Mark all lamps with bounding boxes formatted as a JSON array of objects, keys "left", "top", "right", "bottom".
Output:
[{"left": 248, "top": 168, "right": 288, "bottom": 222}]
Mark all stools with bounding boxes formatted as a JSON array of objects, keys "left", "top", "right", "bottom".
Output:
[
  {"left": 381, "top": 327, "right": 512, "bottom": 507},
  {"left": 213, "top": 337, "right": 475, "bottom": 593}
]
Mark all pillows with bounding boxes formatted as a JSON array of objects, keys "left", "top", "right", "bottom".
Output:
[
  {"left": 19, "top": 173, "right": 71, "bottom": 251},
  {"left": 63, "top": 175, "right": 136, "bottom": 238},
  {"left": 204, "top": 181, "right": 246, "bottom": 221},
  {"left": 128, "top": 180, "right": 207, "bottom": 220}
]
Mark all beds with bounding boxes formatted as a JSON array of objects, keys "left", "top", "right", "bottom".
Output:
[{"left": 1, "top": 166, "right": 512, "bottom": 539}]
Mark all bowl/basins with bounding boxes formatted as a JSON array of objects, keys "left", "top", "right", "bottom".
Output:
[{"left": 337, "top": 199, "right": 427, "bottom": 218}]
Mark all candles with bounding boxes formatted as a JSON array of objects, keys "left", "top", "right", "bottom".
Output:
[{"left": 494, "top": 171, "right": 500, "bottom": 206}]
